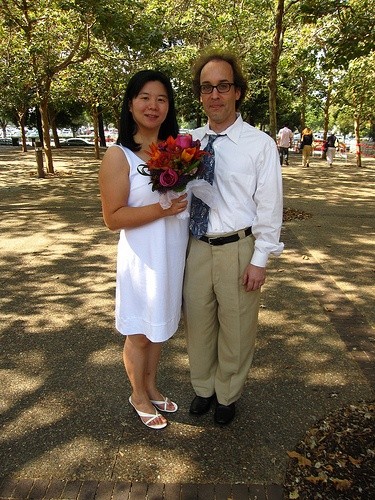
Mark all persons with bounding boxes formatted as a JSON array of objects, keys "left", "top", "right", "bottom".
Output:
[
  {"left": 99, "top": 70, "right": 192, "bottom": 429},
  {"left": 321, "top": 131, "right": 340, "bottom": 169},
  {"left": 301, "top": 128, "right": 314, "bottom": 168},
  {"left": 275, "top": 122, "right": 293, "bottom": 167},
  {"left": 183, "top": 53, "right": 285, "bottom": 426}
]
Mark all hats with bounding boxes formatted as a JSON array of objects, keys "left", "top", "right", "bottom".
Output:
[
  {"left": 301, "top": 128, "right": 312, "bottom": 135},
  {"left": 331, "top": 131, "right": 334, "bottom": 135}
]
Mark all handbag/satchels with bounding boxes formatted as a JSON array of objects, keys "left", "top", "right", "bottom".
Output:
[
  {"left": 334, "top": 138, "right": 339, "bottom": 148},
  {"left": 297, "top": 143, "right": 304, "bottom": 150}
]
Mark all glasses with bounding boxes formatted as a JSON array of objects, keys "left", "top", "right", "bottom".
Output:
[{"left": 199, "top": 83, "right": 235, "bottom": 94}]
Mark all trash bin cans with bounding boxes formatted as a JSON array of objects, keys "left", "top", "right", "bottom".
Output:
[{"left": 11, "top": 137, "right": 20, "bottom": 146}]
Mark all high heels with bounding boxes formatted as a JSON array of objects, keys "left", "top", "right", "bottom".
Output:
[
  {"left": 149, "top": 394, "right": 178, "bottom": 413},
  {"left": 128, "top": 396, "right": 168, "bottom": 430}
]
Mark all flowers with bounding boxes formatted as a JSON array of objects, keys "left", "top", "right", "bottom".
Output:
[{"left": 137, "top": 132, "right": 213, "bottom": 195}]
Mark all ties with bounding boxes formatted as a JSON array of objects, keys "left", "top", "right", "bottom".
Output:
[{"left": 189, "top": 134, "right": 217, "bottom": 240}]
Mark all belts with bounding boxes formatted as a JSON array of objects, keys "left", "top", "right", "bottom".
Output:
[{"left": 199, "top": 227, "right": 252, "bottom": 245}]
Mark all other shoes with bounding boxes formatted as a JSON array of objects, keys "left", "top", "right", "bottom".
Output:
[
  {"left": 190, "top": 396, "right": 212, "bottom": 416},
  {"left": 285, "top": 160, "right": 288, "bottom": 165},
  {"left": 330, "top": 163, "right": 332, "bottom": 167},
  {"left": 214, "top": 402, "right": 235, "bottom": 427},
  {"left": 307, "top": 163, "right": 309, "bottom": 167}
]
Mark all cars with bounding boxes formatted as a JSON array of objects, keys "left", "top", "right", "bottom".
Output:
[{"left": 60, "top": 139, "right": 95, "bottom": 146}]
